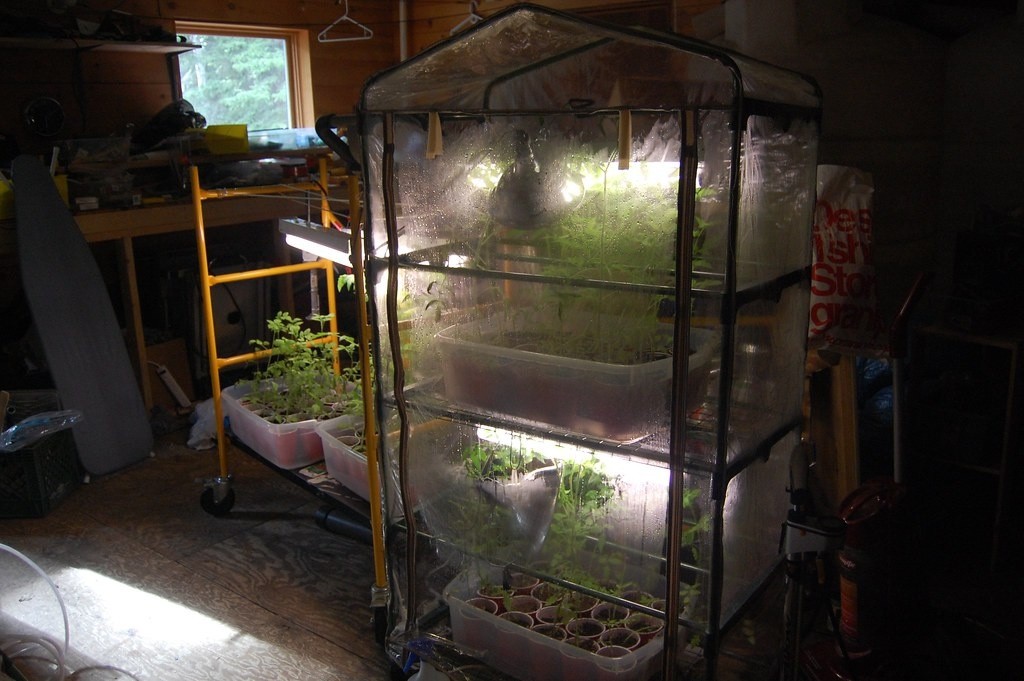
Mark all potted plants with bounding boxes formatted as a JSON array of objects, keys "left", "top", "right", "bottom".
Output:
[
  {"left": 221, "top": 311, "right": 432, "bottom": 511},
  {"left": 433, "top": 140, "right": 722, "bottom": 446},
  {"left": 442, "top": 443, "right": 694, "bottom": 681}
]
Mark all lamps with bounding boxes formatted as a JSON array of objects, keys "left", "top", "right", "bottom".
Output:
[{"left": 487, "top": 131, "right": 586, "bottom": 230}]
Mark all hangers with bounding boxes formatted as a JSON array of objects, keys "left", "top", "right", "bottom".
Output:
[
  {"left": 449, "top": 0, "right": 484, "bottom": 37},
  {"left": 318, "top": 0, "right": 373, "bottom": 42}
]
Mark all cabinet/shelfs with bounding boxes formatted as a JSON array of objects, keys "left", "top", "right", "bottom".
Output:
[
  {"left": 907, "top": 310, "right": 1024, "bottom": 576},
  {"left": 360, "top": 0, "right": 823, "bottom": 681}
]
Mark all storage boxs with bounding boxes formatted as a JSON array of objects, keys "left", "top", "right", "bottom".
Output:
[
  {"left": 0, "top": 428, "right": 77, "bottom": 519},
  {"left": 120, "top": 326, "right": 196, "bottom": 412}
]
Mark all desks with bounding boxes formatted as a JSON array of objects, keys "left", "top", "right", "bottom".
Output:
[{"left": 71, "top": 170, "right": 378, "bottom": 418}]
